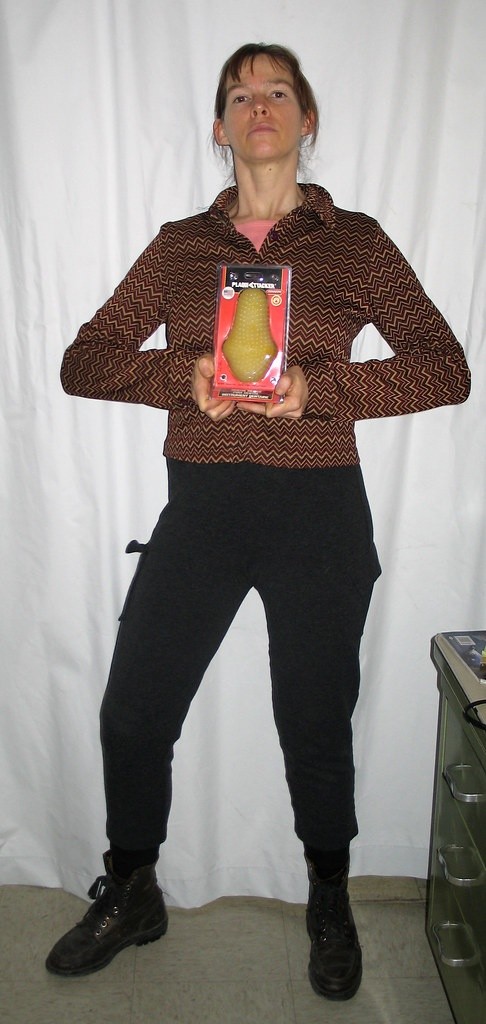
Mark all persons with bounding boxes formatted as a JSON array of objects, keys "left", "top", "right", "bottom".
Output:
[{"left": 44, "top": 43, "right": 472, "bottom": 1001}]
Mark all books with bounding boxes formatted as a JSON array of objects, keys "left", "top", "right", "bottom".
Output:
[{"left": 441, "top": 633, "right": 486, "bottom": 684}]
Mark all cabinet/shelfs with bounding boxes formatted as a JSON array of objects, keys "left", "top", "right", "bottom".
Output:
[{"left": 426, "top": 674, "right": 486, "bottom": 1024}]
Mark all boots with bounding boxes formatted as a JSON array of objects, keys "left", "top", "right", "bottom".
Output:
[
  {"left": 46, "top": 850, "right": 167, "bottom": 976},
  {"left": 304, "top": 844, "right": 362, "bottom": 1000}
]
